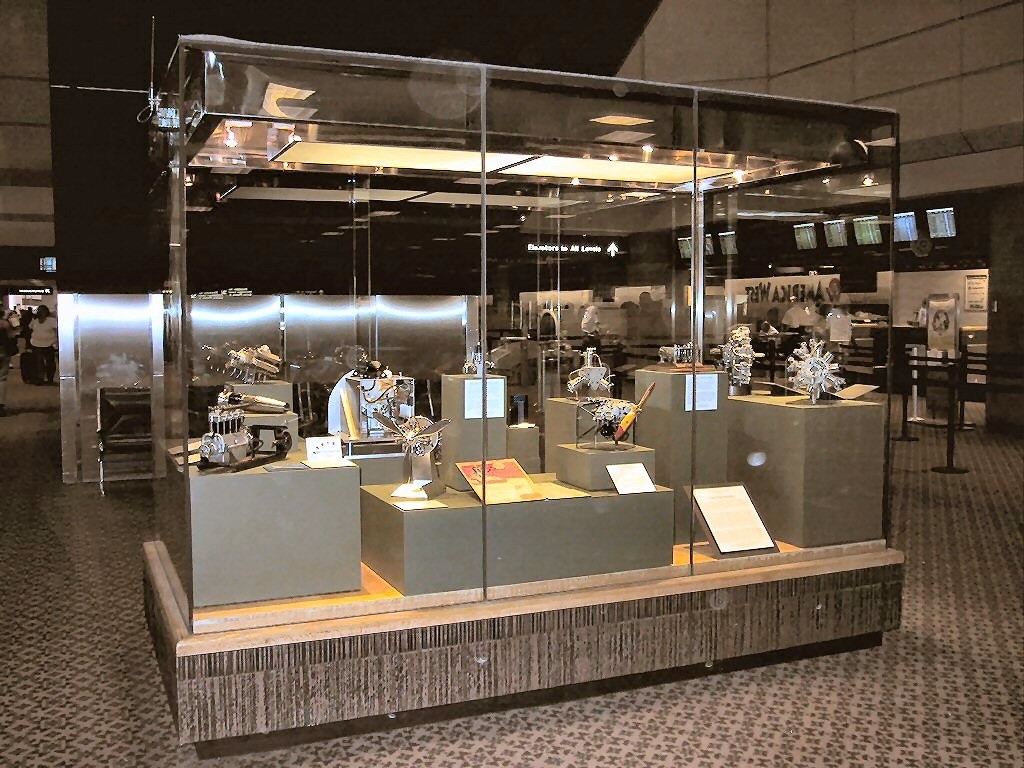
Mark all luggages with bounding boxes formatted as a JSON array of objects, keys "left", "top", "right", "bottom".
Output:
[{"left": 20, "top": 348, "right": 44, "bottom": 383}]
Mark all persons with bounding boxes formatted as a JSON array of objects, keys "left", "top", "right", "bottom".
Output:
[
  {"left": 580, "top": 291, "right": 664, "bottom": 361},
  {"left": 780, "top": 296, "right": 852, "bottom": 355},
  {"left": 762, "top": 322, "right": 778, "bottom": 336},
  {"left": 0, "top": 304, "right": 57, "bottom": 415}
]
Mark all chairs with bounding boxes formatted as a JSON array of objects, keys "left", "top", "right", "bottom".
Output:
[
  {"left": 96, "top": 386, "right": 150, "bottom": 497},
  {"left": 187, "top": 385, "right": 224, "bottom": 437},
  {"left": 292, "top": 382, "right": 335, "bottom": 437},
  {"left": 426, "top": 379, "right": 441, "bottom": 419}
]
